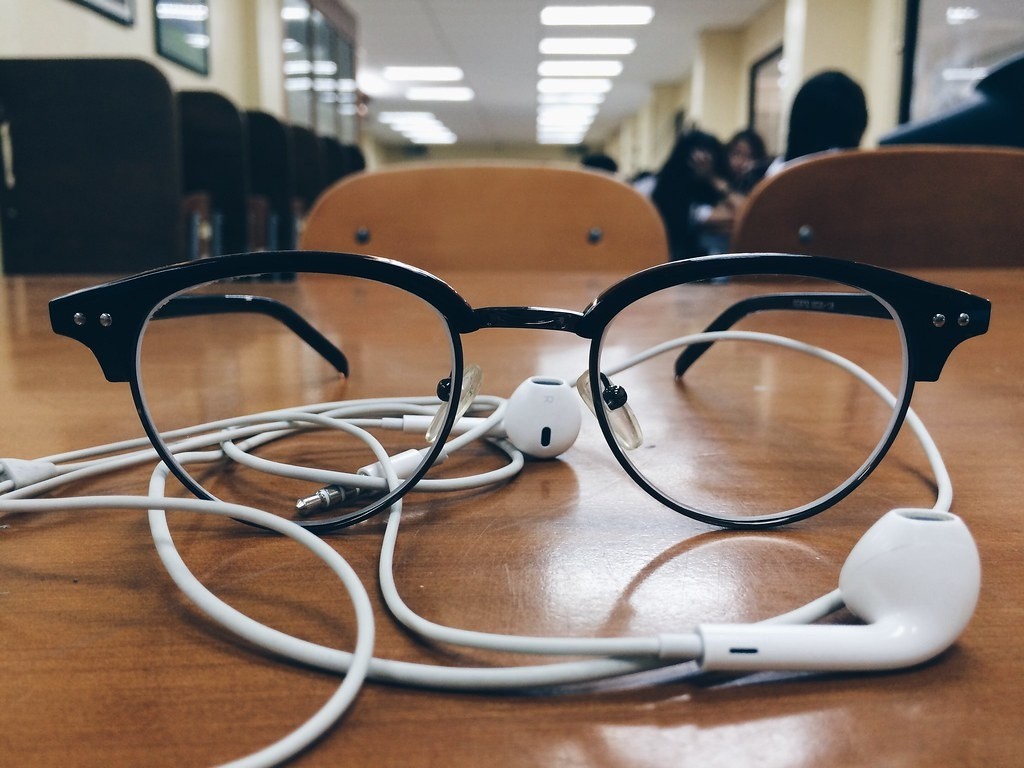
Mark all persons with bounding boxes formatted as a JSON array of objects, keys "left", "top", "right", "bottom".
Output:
[{"left": 582, "top": 69, "right": 868, "bottom": 256}]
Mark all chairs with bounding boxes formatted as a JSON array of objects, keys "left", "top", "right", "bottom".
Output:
[
  {"left": 298, "top": 161, "right": 671, "bottom": 279},
  {"left": 0, "top": 59, "right": 367, "bottom": 280},
  {"left": 727, "top": 147, "right": 1024, "bottom": 281}
]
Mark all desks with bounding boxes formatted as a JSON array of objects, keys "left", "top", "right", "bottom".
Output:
[{"left": 0, "top": 274, "right": 1024, "bottom": 767}]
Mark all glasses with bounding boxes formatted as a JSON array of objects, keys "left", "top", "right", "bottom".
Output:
[{"left": 49, "top": 250, "right": 992, "bottom": 537}]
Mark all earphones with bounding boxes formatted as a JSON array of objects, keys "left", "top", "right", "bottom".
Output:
[
  {"left": 404, "top": 375, "right": 581, "bottom": 459},
  {"left": 700, "top": 508, "right": 984, "bottom": 672}
]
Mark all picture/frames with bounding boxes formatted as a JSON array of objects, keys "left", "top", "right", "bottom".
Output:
[{"left": 71, "top": 0, "right": 210, "bottom": 77}]
